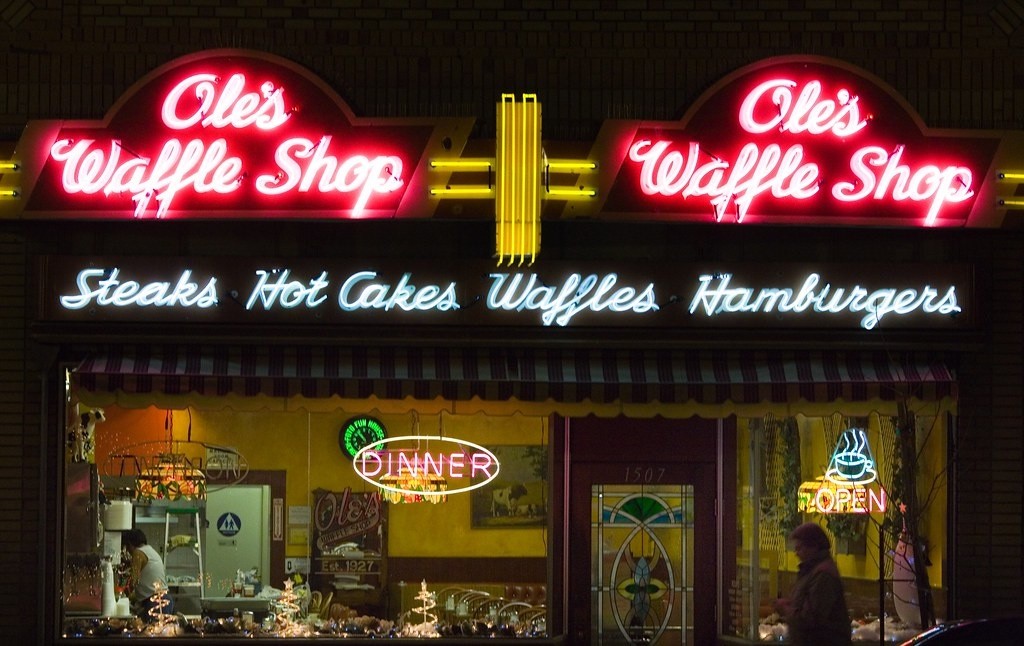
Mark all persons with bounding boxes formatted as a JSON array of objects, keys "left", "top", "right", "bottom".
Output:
[
  {"left": 779, "top": 523, "right": 852, "bottom": 646},
  {"left": 122, "top": 529, "right": 173, "bottom": 625}
]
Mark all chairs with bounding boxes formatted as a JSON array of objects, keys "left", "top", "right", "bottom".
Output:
[
  {"left": 319, "top": 591, "right": 334, "bottom": 620},
  {"left": 309, "top": 590, "right": 322, "bottom": 613}
]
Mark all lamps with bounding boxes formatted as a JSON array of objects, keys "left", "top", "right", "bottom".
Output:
[
  {"left": 376, "top": 411, "right": 449, "bottom": 504},
  {"left": 793, "top": 413, "right": 870, "bottom": 515},
  {"left": 134, "top": 408, "right": 208, "bottom": 501}
]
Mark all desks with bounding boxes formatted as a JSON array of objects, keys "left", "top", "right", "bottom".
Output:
[{"left": 201, "top": 597, "right": 271, "bottom": 612}]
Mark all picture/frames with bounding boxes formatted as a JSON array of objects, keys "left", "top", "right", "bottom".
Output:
[{"left": 470, "top": 443, "right": 547, "bottom": 529}]
